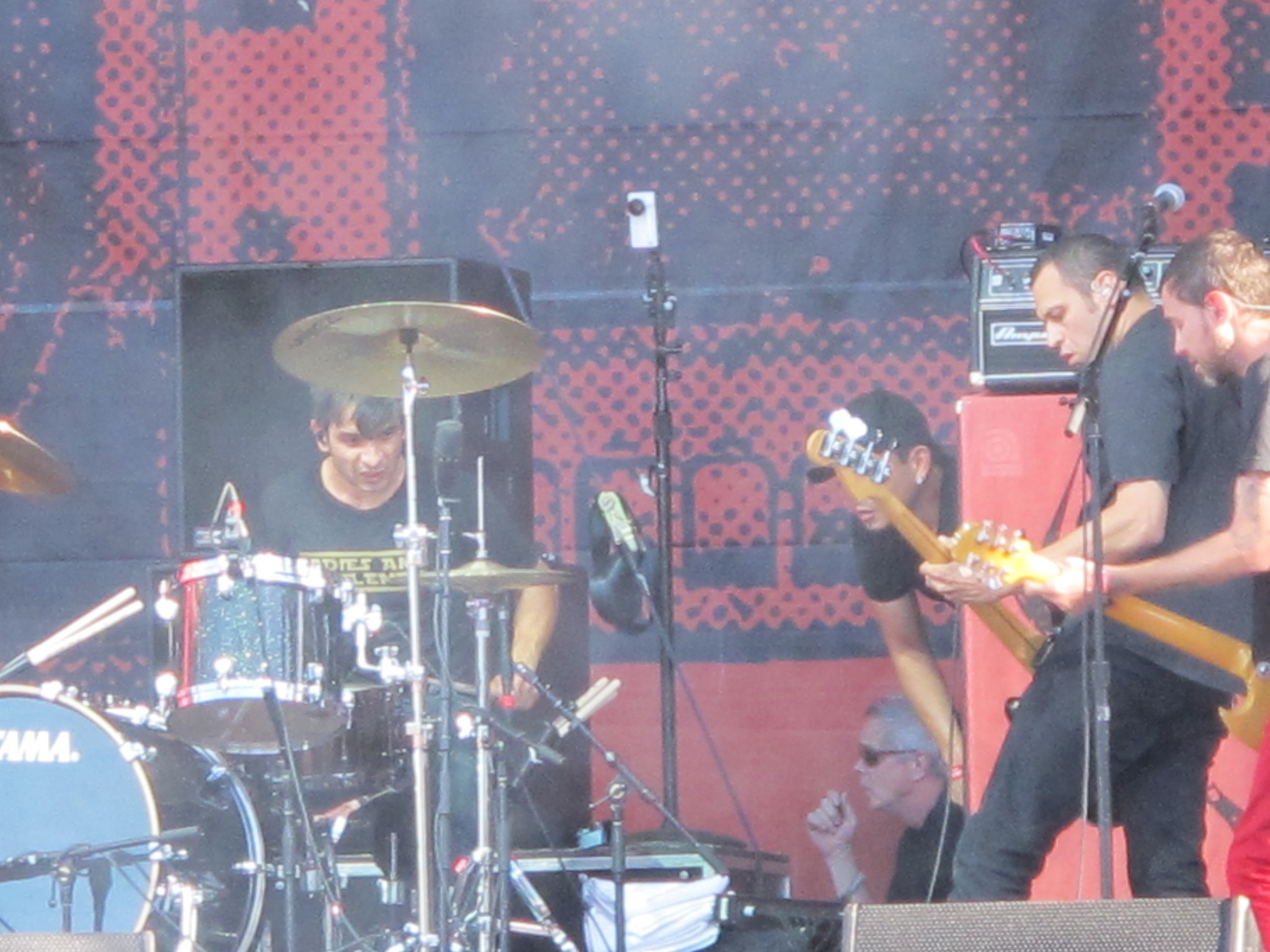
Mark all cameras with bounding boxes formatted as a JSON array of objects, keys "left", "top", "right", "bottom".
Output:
[{"left": 626, "top": 188, "right": 663, "bottom": 253}]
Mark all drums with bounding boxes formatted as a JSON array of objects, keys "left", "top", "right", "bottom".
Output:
[
  {"left": 1, "top": 682, "right": 267, "bottom": 951},
  {"left": 163, "top": 552, "right": 350, "bottom": 760}
]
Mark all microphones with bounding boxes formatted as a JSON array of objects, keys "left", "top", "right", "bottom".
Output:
[{"left": 1146, "top": 178, "right": 1183, "bottom": 238}]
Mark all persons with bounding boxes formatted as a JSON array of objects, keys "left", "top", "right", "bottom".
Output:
[
  {"left": 245, "top": 389, "right": 561, "bottom": 951},
  {"left": 802, "top": 696, "right": 966, "bottom": 904},
  {"left": 1021, "top": 230, "right": 1270, "bottom": 952},
  {"left": 916, "top": 234, "right": 1262, "bottom": 902},
  {"left": 823, "top": 390, "right": 968, "bottom": 805}
]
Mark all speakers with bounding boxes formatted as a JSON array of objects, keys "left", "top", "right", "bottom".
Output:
[{"left": 842, "top": 896, "right": 1265, "bottom": 952}]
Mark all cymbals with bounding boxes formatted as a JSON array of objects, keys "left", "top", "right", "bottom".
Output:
[
  {"left": 388, "top": 559, "right": 571, "bottom": 598},
  {"left": 0, "top": 415, "right": 73, "bottom": 498},
  {"left": 270, "top": 301, "right": 544, "bottom": 400}
]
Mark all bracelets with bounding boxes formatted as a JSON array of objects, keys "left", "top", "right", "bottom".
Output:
[{"left": 838, "top": 871, "right": 866, "bottom": 901}]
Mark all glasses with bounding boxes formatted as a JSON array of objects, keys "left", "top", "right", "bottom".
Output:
[{"left": 857, "top": 740, "right": 924, "bottom": 767}]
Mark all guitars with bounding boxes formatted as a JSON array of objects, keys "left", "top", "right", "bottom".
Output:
[
  {"left": 949, "top": 518, "right": 1270, "bottom": 756},
  {"left": 807, "top": 405, "right": 1123, "bottom": 826}
]
